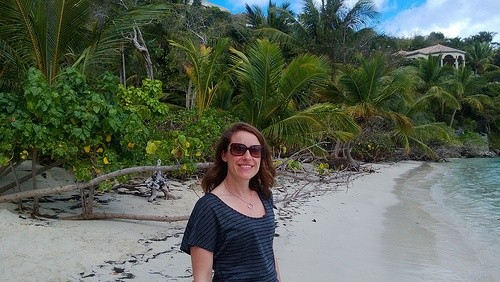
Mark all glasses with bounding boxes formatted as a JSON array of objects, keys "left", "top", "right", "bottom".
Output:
[{"left": 226, "top": 143, "right": 264, "bottom": 158}]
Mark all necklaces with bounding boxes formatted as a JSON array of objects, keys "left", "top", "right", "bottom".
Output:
[{"left": 223, "top": 180, "right": 254, "bottom": 210}]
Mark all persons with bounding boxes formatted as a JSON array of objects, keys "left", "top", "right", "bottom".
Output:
[{"left": 179, "top": 121, "right": 283, "bottom": 282}]
